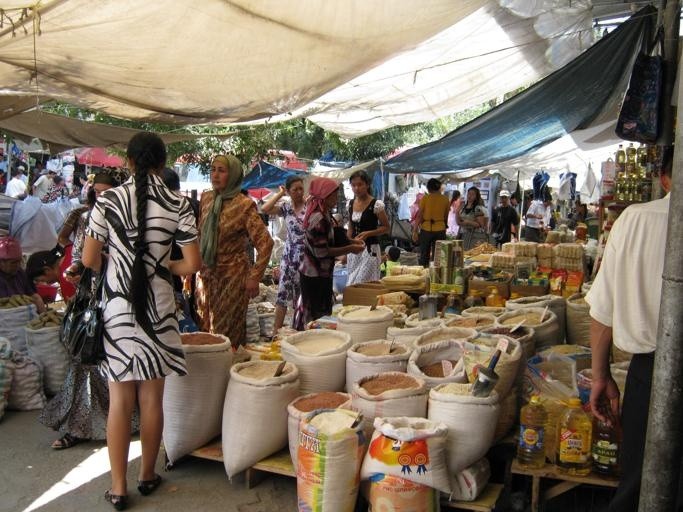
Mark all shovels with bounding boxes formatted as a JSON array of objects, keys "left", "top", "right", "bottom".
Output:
[
  {"left": 472, "top": 349, "right": 502, "bottom": 397},
  {"left": 419, "top": 276, "right": 438, "bottom": 322}
]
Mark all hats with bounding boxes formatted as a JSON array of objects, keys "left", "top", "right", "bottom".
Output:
[{"left": 498, "top": 189, "right": 512, "bottom": 198}]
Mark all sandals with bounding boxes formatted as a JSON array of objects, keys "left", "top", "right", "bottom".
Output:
[
  {"left": 50, "top": 432, "right": 77, "bottom": 452},
  {"left": 136, "top": 474, "right": 162, "bottom": 496},
  {"left": 104, "top": 488, "right": 127, "bottom": 510}
]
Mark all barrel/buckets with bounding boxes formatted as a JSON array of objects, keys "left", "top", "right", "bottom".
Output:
[
  {"left": 616, "top": 141, "right": 650, "bottom": 202},
  {"left": 518, "top": 396, "right": 618, "bottom": 476},
  {"left": 333, "top": 268, "right": 348, "bottom": 293},
  {"left": 446, "top": 286, "right": 521, "bottom": 310}
]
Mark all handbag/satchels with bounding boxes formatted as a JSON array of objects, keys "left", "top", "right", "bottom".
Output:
[
  {"left": 330, "top": 214, "right": 350, "bottom": 248},
  {"left": 56, "top": 251, "right": 107, "bottom": 368},
  {"left": 613, "top": 23, "right": 665, "bottom": 145}
]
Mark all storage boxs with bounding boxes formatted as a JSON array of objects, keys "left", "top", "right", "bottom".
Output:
[
  {"left": 508, "top": 277, "right": 549, "bottom": 297},
  {"left": 466, "top": 273, "right": 514, "bottom": 300},
  {"left": 341, "top": 283, "right": 388, "bottom": 311}
]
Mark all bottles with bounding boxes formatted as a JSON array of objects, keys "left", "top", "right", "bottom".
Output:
[
  {"left": 613, "top": 140, "right": 648, "bottom": 202},
  {"left": 447, "top": 289, "right": 518, "bottom": 314},
  {"left": 431, "top": 288, "right": 443, "bottom": 312},
  {"left": 514, "top": 394, "right": 591, "bottom": 478},
  {"left": 593, "top": 398, "right": 620, "bottom": 477}
]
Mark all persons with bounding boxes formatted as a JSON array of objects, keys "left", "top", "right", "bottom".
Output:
[
  {"left": 5, "top": 154, "right": 274, "bottom": 449},
  {"left": 82, "top": 131, "right": 202, "bottom": 508},
  {"left": 261, "top": 169, "right": 401, "bottom": 343},
  {"left": 584, "top": 147, "right": 683, "bottom": 512},
  {"left": 409, "top": 178, "right": 588, "bottom": 267}
]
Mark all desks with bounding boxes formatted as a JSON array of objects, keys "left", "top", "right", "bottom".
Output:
[{"left": 509, "top": 459, "right": 621, "bottom": 512}]
